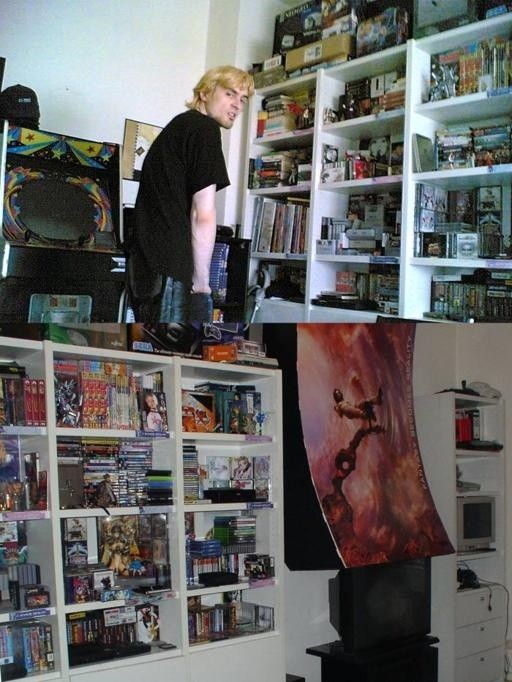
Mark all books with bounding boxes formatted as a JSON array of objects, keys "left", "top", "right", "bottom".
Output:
[
  {"left": 429, "top": 36, "right": 511, "bottom": 101},
  {"left": 321, "top": 133, "right": 404, "bottom": 180},
  {"left": 257, "top": 151, "right": 306, "bottom": 186},
  {"left": 181, "top": 382, "right": 262, "bottom": 435},
  {"left": 119, "top": 438, "right": 174, "bottom": 506},
  {"left": 413, "top": 182, "right": 503, "bottom": 260},
  {"left": 57, "top": 436, "right": 119, "bottom": 473},
  {"left": 185, "top": 512, "right": 276, "bottom": 585},
  {"left": 54, "top": 359, "right": 169, "bottom": 431},
  {"left": 66, "top": 518, "right": 87, "bottom": 540},
  {"left": 412, "top": 123, "right": 512, "bottom": 171},
  {"left": 188, "top": 591, "right": 275, "bottom": 643},
  {"left": 430, "top": 269, "right": 512, "bottom": 325},
  {"left": 261, "top": 89, "right": 316, "bottom": 136},
  {"left": 311, "top": 271, "right": 398, "bottom": 314},
  {"left": 66, "top": 604, "right": 160, "bottom": 652},
  {"left": 251, "top": 197, "right": 311, "bottom": 254},
  {"left": 0, "top": 618, "right": 55, "bottom": 672},
  {"left": 333, "top": 66, "right": 406, "bottom": 110},
  {"left": 80, "top": 472, "right": 105, "bottom": 483},
  {"left": 79, "top": 482, "right": 99, "bottom": 509},
  {"left": 139, "top": 514, "right": 169, "bottom": 591},
  {"left": 87, "top": 566, "right": 115, "bottom": 601},
  {"left": 183, "top": 440, "right": 272, "bottom": 505},
  {"left": 1, "top": 436, "right": 49, "bottom": 511},
  {"left": 110, "top": 472, "right": 118, "bottom": 500},
  {"left": 0, "top": 362, "right": 48, "bottom": 427},
  {"left": 0, "top": 520, "right": 51, "bottom": 610},
  {"left": 62, "top": 540, "right": 93, "bottom": 604}
]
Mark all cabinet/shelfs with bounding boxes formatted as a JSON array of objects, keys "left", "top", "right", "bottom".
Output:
[
  {"left": 239, "top": 11, "right": 512, "bottom": 324},
  {"left": 1, "top": 333, "right": 283, "bottom": 682},
  {"left": 417, "top": 393, "right": 509, "bottom": 679}
]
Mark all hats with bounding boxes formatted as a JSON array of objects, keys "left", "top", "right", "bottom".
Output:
[{"left": 0, "top": 83, "right": 42, "bottom": 128}]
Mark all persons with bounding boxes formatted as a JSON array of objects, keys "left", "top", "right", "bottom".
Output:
[
  {"left": 94, "top": 472, "right": 118, "bottom": 508},
  {"left": 126, "top": 65, "right": 255, "bottom": 323},
  {"left": 101, "top": 520, "right": 142, "bottom": 578}
]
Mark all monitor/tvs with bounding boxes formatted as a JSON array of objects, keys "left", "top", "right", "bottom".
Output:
[
  {"left": 328, "top": 557, "right": 433, "bottom": 642},
  {"left": 457, "top": 495, "right": 497, "bottom": 552}
]
